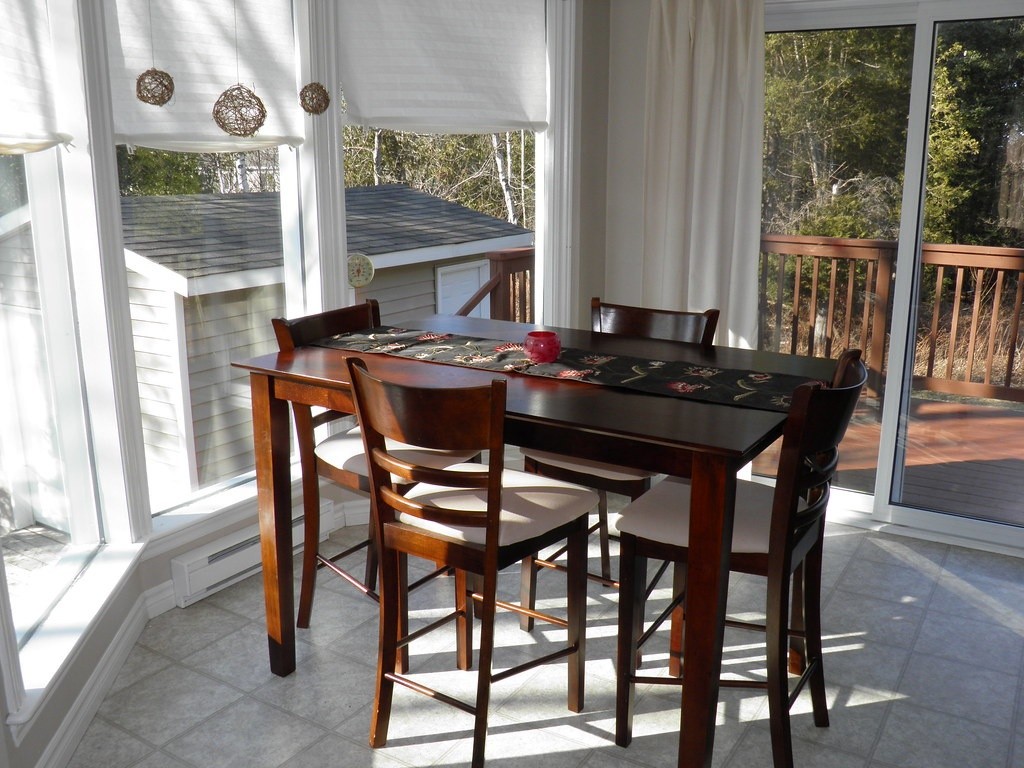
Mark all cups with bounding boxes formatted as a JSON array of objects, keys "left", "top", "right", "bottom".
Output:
[{"left": 523, "top": 330, "right": 560, "bottom": 365}]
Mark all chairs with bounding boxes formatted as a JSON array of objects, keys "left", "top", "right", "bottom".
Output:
[{"left": 272, "top": 291, "right": 867, "bottom": 768}]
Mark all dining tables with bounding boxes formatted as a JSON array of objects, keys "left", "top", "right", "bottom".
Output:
[{"left": 231, "top": 312, "right": 836, "bottom": 768}]
